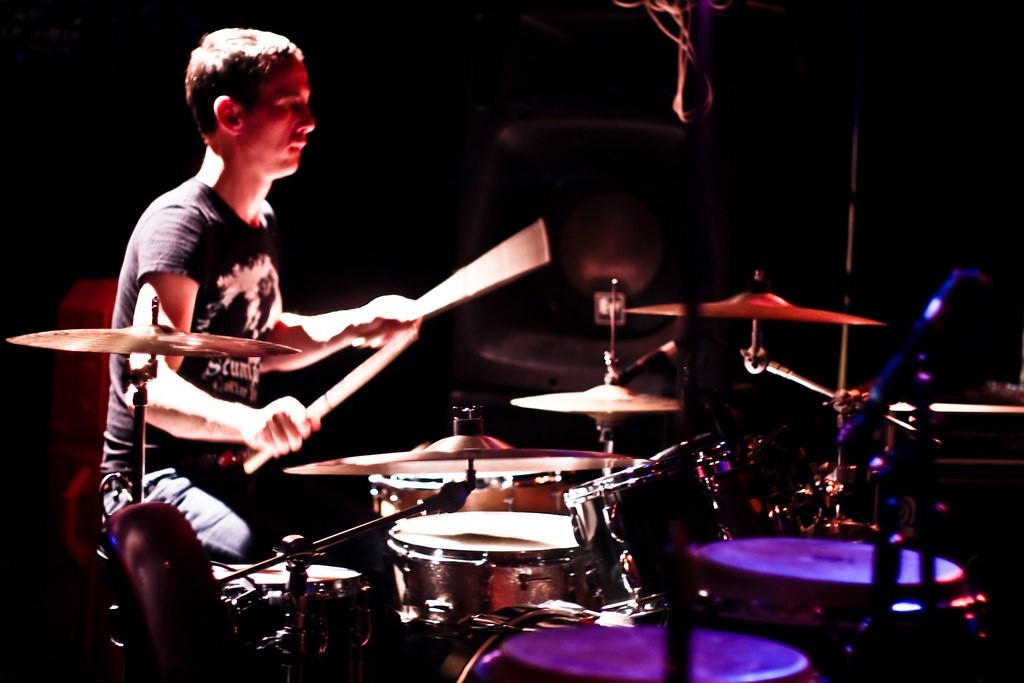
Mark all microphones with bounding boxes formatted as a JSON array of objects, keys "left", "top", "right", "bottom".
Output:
[
  {"left": 611, "top": 341, "right": 683, "bottom": 388},
  {"left": 836, "top": 272, "right": 965, "bottom": 455}
]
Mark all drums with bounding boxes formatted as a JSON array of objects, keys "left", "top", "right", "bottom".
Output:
[
  {"left": 369, "top": 472, "right": 581, "bottom": 516},
  {"left": 433, "top": 599, "right": 635, "bottom": 683},
  {"left": 562, "top": 440, "right": 762, "bottom": 604},
  {"left": 212, "top": 563, "right": 362, "bottom": 683},
  {"left": 387, "top": 511, "right": 602, "bottom": 643},
  {"left": 682, "top": 537, "right": 990, "bottom": 683},
  {"left": 472, "top": 627, "right": 828, "bottom": 683}
]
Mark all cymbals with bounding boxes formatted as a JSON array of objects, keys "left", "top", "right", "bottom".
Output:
[
  {"left": 622, "top": 291, "right": 887, "bottom": 325},
  {"left": 5, "top": 324, "right": 302, "bottom": 357},
  {"left": 282, "top": 434, "right": 660, "bottom": 478},
  {"left": 510, "top": 384, "right": 681, "bottom": 424}
]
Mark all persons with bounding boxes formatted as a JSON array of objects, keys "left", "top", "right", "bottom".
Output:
[{"left": 103, "top": 28, "right": 423, "bottom": 565}]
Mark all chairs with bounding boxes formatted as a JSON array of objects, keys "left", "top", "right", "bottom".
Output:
[
  {"left": 449, "top": 114, "right": 703, "bottom": 437},
  {"left": 46, "top": 275, "right": 124, "bottom": 683}
]
